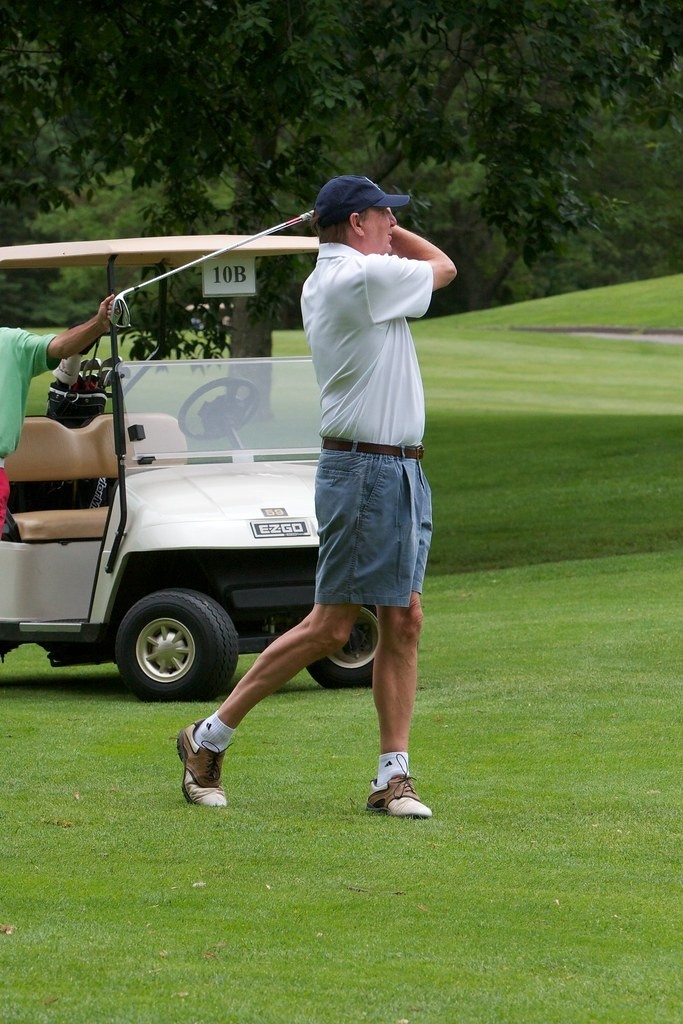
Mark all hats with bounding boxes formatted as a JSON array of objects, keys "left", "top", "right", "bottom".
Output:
[{"left": 315, "top": 175, "right": 410, "bottom": 228}]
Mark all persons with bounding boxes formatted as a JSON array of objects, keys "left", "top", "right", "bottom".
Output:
[
  {"left": 0, "top": 293, "right": 122, "bottom": 542},
  {"left": 176, "top": 176, "right": 457, "bottom": 818}
]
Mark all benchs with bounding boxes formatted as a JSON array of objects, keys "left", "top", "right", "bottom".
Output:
[{"left": 6, "top": 412, "right": 188, "bottom": 546}]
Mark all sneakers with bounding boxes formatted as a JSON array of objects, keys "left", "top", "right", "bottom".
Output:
[
  {"left": 366, "top": 774, "right": 433, "bottom": 818},
  {"left": 177, "top": 719, "right": 228, "bottom": 807}
]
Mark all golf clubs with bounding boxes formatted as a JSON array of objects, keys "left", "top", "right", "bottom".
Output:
[
  {"left": 109, "top": 206, "right": 316, "bottom": 329},
  {"left": 65, "top": 321, "right": 131, "bottom": 392}
]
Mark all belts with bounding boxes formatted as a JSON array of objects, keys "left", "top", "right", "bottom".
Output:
[{"left": 323, "top": 438, "right": 424, "bottom": 459}]
